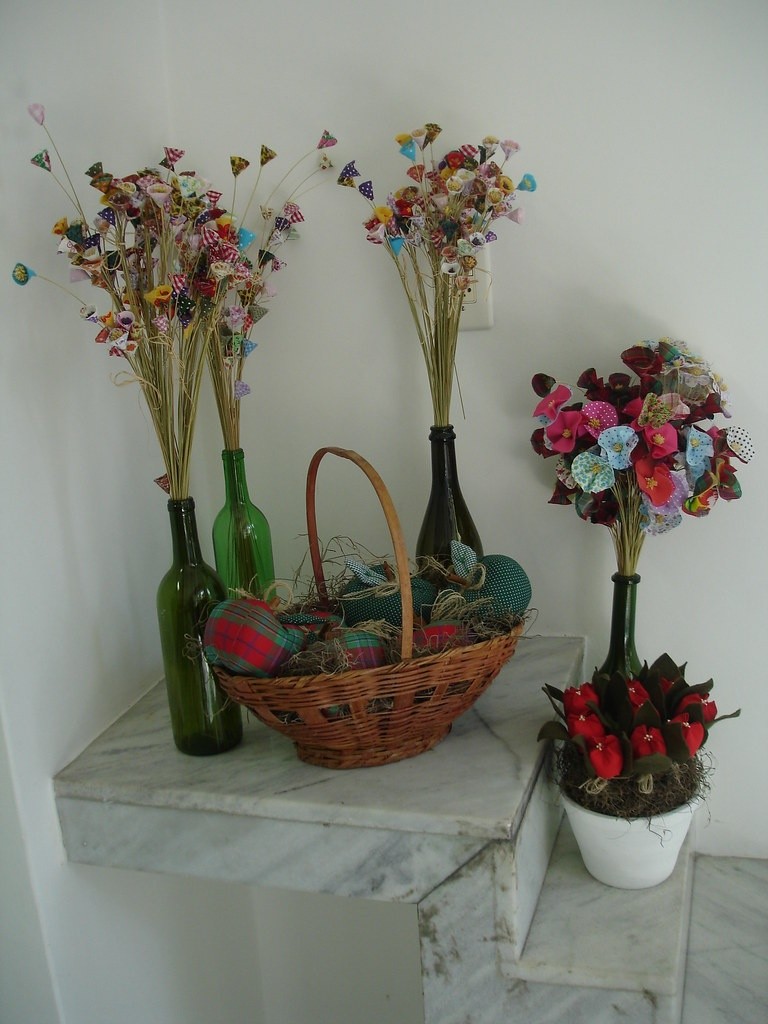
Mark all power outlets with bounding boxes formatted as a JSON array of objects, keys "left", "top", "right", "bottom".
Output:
[
  {"left": 460, "top": 243, "right": 493, "bottom": 333},
  {"left": 457, "top": 240, "right": 492, "bottom": 332}
]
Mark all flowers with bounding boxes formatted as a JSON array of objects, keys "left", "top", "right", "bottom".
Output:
[
  {"left": 11, "top": 103, "right": 338, "bottom": 498},
  {"left": 337, "top": 122, "right": 538, "bottom": 425},
  {"left": 531, "top": 338, "right": 755, "bottom": 575},
  {"left": 537, "top": 653, "right": 741, "bottom": 846}
]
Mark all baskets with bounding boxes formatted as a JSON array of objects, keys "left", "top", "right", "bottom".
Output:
[{"left": 209, "top": 446, "right": 525, "bottom": 770}]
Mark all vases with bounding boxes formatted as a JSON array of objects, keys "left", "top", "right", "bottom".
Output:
[{"left": 559, "top": 784, "right": 703, "bottom": 888}]
